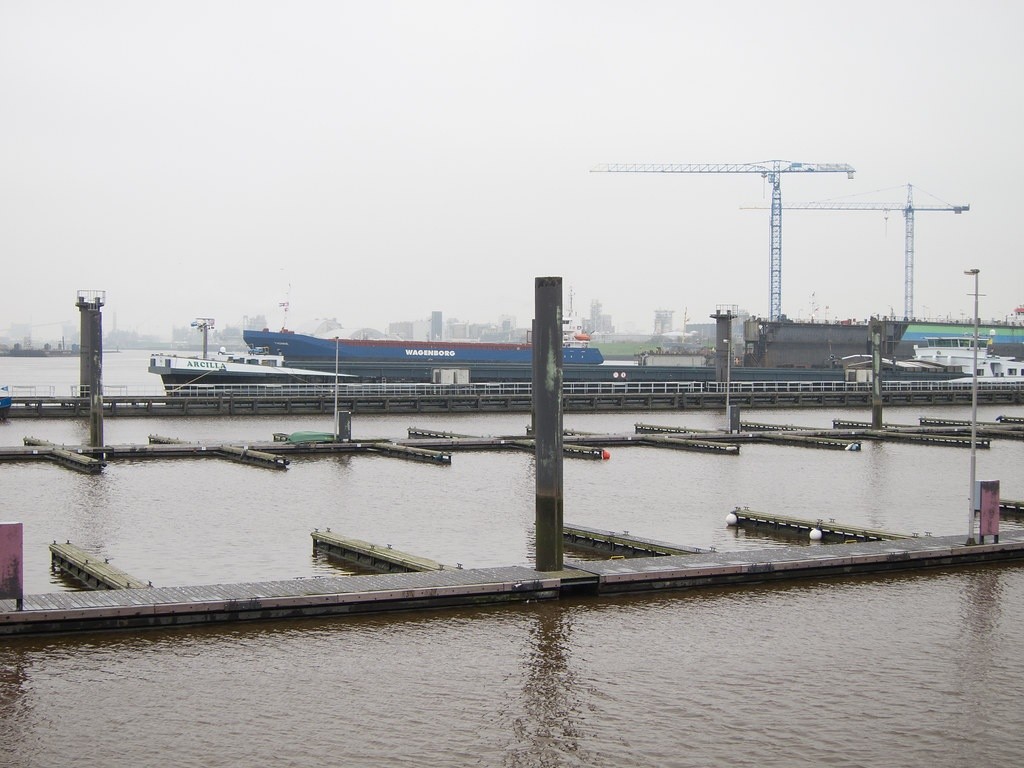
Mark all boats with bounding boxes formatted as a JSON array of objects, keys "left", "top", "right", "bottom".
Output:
[
  {"left": 243, "top": 327, "right": 603, "bottom": 383},
  {"left": 146, "top": 352, "right": 360, "bottom": 398},
  {"left": 912, "top": 335, "right": 1024, "bottom": 389}
]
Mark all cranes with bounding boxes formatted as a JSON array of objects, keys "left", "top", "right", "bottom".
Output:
[
  {"left": 737, "top": 183, "right": 973, "bottom": 322},
  {"left": 589, "top": 157, "right": 855, "bottom": 325}
]
